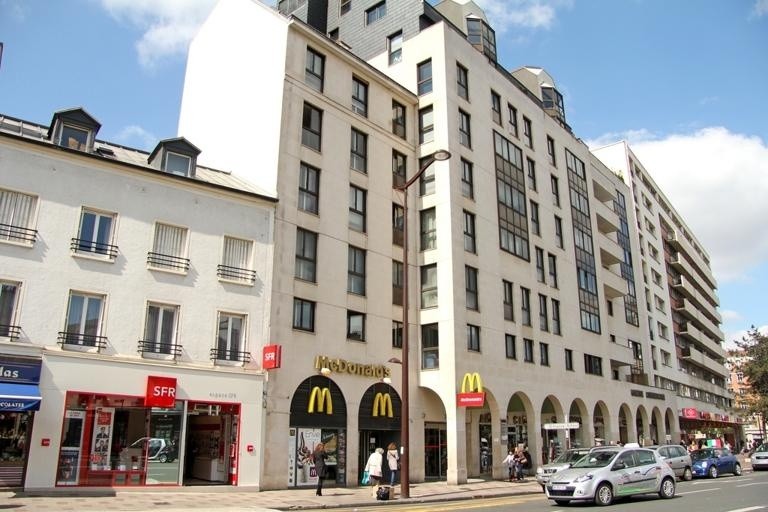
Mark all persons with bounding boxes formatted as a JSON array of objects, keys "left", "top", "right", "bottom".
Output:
[
  {"left": 616, "top": 441, "right": 620, "bottom": 447},
  {"left": 653, "top": 439, "right": 659, "bottom": 445},
  {"left": 746, "top": 439, "right": 760, "bottom": 451},
  {"left": 680, "top": 438, "right": 707, "bottom": 453},
  {"left": 364, "top": 448, "right": 384, "bottom": 499},
  {"left": 387, "top": 441, "right": 399, "bottom": 486},
  {"left": 502, "top": 448, "right": 516, "bottom": 482},
  {"left": 724, "top": 440, "right": 733, "bottom": 453},
  {"left": 95, "top": 440, "right": 108, "bottom": 452},
  {"left": 96, "top": 427, "right": 108, "bottom": 438},
  {"left": 313, "top": 443, "right": 328, "bottom": 496},
  {"left": 514, "top": 447, "right": 524, "bottom": 483}
]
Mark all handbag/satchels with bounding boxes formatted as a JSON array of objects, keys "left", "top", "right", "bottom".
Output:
[
  {"left": 362, "top": 471, "right": 369, "bottom": 485},
  {"left": 397, "top": 463, "right": 401, "bottom": 471}
]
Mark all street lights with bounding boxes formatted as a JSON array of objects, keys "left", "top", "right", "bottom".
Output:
[{"left": 390, "top": 150, "right": 451, "bottom": 498}]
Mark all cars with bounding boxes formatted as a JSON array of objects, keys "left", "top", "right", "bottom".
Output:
[
  {"left": 750, "top": 442, "right": 768, "bottom": 471},
  {"left": 129, "top": 436, "right": 175, "bottom": 463},
  {"left": 535, "top": 443, "right": 742, "bottom": 506}
]
